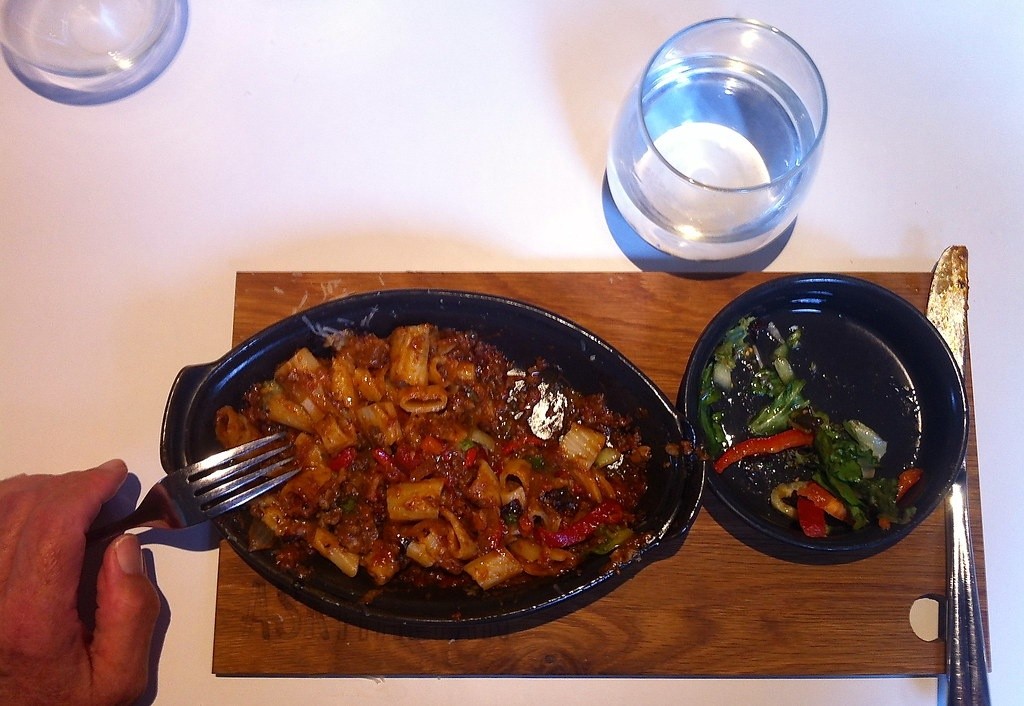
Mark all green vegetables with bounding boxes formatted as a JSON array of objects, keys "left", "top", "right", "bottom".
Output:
[{"left": 697, "top": 315, "right": 916, "bottom": 532}]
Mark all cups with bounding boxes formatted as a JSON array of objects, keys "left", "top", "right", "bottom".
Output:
[
  {"left": 608, "top": 17, "right": 830, "bottom": 261},
  {"left": 0, "top": 0, "right": 180, "bottom": 93}
]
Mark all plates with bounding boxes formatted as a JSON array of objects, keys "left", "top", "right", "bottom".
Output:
[{"left": 684, "top": 274, "right": 969, "bottom": 554}]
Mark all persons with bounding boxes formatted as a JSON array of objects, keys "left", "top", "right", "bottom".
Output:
[{"left": 0, "top": 459, "right": 161, "bottom": 706}]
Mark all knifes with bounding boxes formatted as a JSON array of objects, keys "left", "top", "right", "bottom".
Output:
[{"left": 922, "top": 247, "right": 989, "bottom": 706}]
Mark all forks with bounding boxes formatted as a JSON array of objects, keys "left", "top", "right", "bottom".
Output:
[{"left": 84, "top": 430, "right": 301, "bottom": 547}]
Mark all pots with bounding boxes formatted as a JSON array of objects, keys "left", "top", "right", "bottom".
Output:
[{"left": 160, "top": 286, "right": 704, "bottom": 627}]
